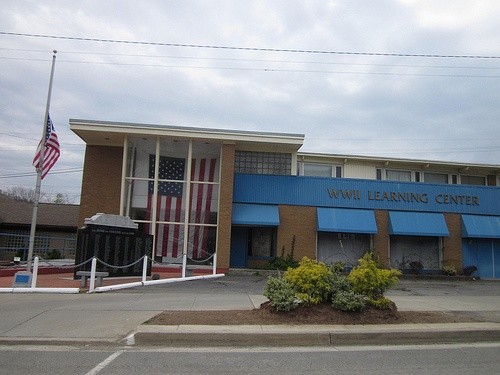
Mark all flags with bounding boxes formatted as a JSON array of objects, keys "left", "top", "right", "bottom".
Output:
[
  {"left": 32, "top": 113, "right": 61, "bottom": 180},
  {"left": 143, "top": 153, "right": 217, "bottom": 259}
]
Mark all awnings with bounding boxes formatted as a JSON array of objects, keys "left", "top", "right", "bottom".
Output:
[
  {"left": 230, "top": 203, "right": 280, "bottom": 228},
  {"left": 316, "top": 207, "right": 379, "bottom": 235},
  {"left": 388, "top": 211, "right": 450, "bottom": 237},
  {"left": 460, "top": 214, "right": 500, "bottom": 239}
]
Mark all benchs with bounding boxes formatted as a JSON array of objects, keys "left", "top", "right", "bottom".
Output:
[{"left": 74, "top": 269, "right": 110, "bottom": 289}]
